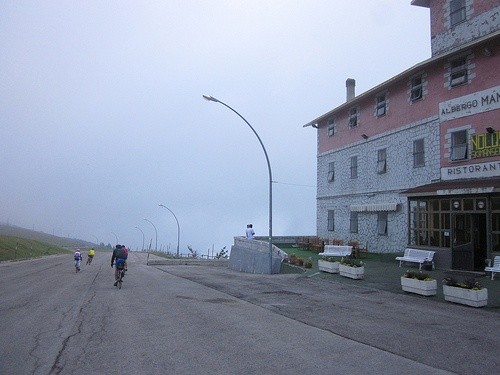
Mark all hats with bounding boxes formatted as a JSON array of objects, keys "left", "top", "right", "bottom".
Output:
[
  {"left": 121, "top": 245, "right": 125, "bottom": 247},
  {"left": 92, "top": 248, "right": 94, "bottom": 250},
  {"left": 116, "top": 245, "right": 121, "bottom": 248},
  {"left": 77, "top": 249, "right": 80, "bottom": 251}
]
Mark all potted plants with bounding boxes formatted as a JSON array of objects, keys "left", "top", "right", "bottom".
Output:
[
  {"left": 338, "top": 257, "right": 365, "bottom": 280},
  {"left": 317, "top": 255, "right": 339, "bottom": 274},
  {"left": 442, "top": 270, "right": 489, "bottom": 309},
  {"left": 400, "top": 268, "right": 439, "bottom": 297},
  {"left": 295, "top": 256, "right": 303, "bottom": 266},
  {"left": 304, "top": 257, "right": 312, "bottom": 267},
  {"left": 288, "top": 252, "right": 297, "bottom": 264}
]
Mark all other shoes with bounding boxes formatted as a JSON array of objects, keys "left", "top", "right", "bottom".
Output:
[
  {"left": 121, "top": 278, "right": 124, "bottom": 282},
  {"left": 114, "top": 281, "right": 118, "bottom": 286},
  {"left": 126, "top": 269, "right": 127, "bottom": 271}
]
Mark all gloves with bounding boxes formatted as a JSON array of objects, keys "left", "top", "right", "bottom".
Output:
[{"left": 111, "top": 264, "right": 113, "bottom": 268}]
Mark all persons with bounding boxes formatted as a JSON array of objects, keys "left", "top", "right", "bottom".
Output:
[
  {"left": 87, "top": 248, "right": 95, "bottom": 263},
  {"left": 74, "top": 249, "right": 82, "bottom": 271},
  {"left": 246, "top": 224, "right": 255, "bottom": 239},
  {"left": 111, "top": 244, "right": 128, "bottom": 286}
]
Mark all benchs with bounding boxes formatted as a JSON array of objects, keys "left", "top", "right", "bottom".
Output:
[
  {"left": 318, "top": 244, "right": 354, "bottom": 259},
  {"left": 484, "top": 256, "right": 500, "bottom": 281},
  {"left": 394, "top": 247, "right": 436, "bottom": 270}
]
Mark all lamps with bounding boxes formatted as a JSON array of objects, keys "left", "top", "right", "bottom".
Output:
[
  {"left": 360, "top": 134, "right": 368, "bottom": 139},
  {"left": 485, "top": 127, "right": 495, "bottom": 134}
]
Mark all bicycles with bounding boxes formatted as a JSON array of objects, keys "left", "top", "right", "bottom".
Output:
[
  {"left": 74, "top": 257, "right": 82, "bottom": 272},
  {"left": 86, "top": 255, "right": 93, "bottom": 265},
  {"left": 111, "top": 263, "right": 127, "bottom": 289}
]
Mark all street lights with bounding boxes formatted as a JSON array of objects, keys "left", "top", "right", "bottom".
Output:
[
  {"left": 136, "top": 226, "right": 144, "bottom": 253},
  {"left": 202, "top": 94, "right": 273, "bottom": 275},
  {"left": 144, "top": 218, "right": 158, "bottom": 252},
  {"left": 158, "top": 203, "right": 180, "bottom": 259}
]
